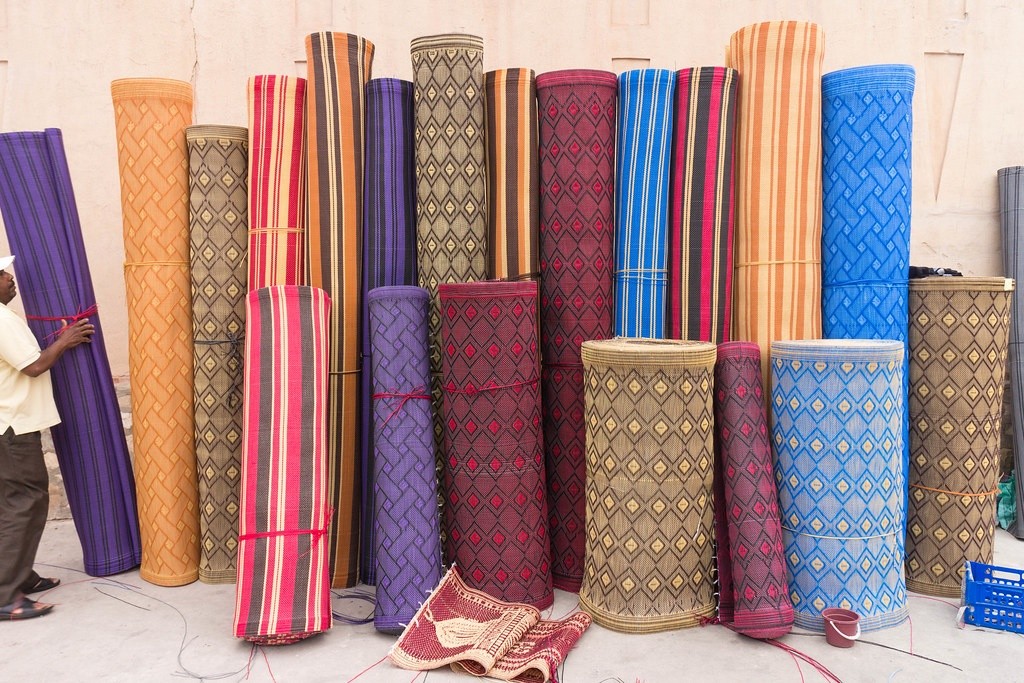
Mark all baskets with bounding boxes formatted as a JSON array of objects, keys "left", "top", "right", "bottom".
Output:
[{"left": 963, "top": 560, "right": 1024, "bottom": 634}]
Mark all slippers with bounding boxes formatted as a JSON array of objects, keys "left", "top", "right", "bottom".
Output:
[
  {"left": 22, "top": 577, "right": 61, "bottom": 594},
  {"left": 0, "top": 597, "right": 55, "bottom": 620}
]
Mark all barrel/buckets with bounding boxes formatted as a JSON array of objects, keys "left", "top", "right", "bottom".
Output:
[{"left": 822, "top": 608, "right": 862, "bottom": 648}]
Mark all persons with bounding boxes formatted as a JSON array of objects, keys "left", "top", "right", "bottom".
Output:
[{"left": 0, "top": 255, "right": 95, "bottom": 620}]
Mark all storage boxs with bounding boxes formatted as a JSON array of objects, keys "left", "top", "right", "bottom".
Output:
[{"left": 963, "top": 561, "right": 1024, "bottom": 635}]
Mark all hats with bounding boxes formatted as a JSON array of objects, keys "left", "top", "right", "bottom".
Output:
[{"left": 0, "top": 255, "right": 16, "bottom": 273}]
